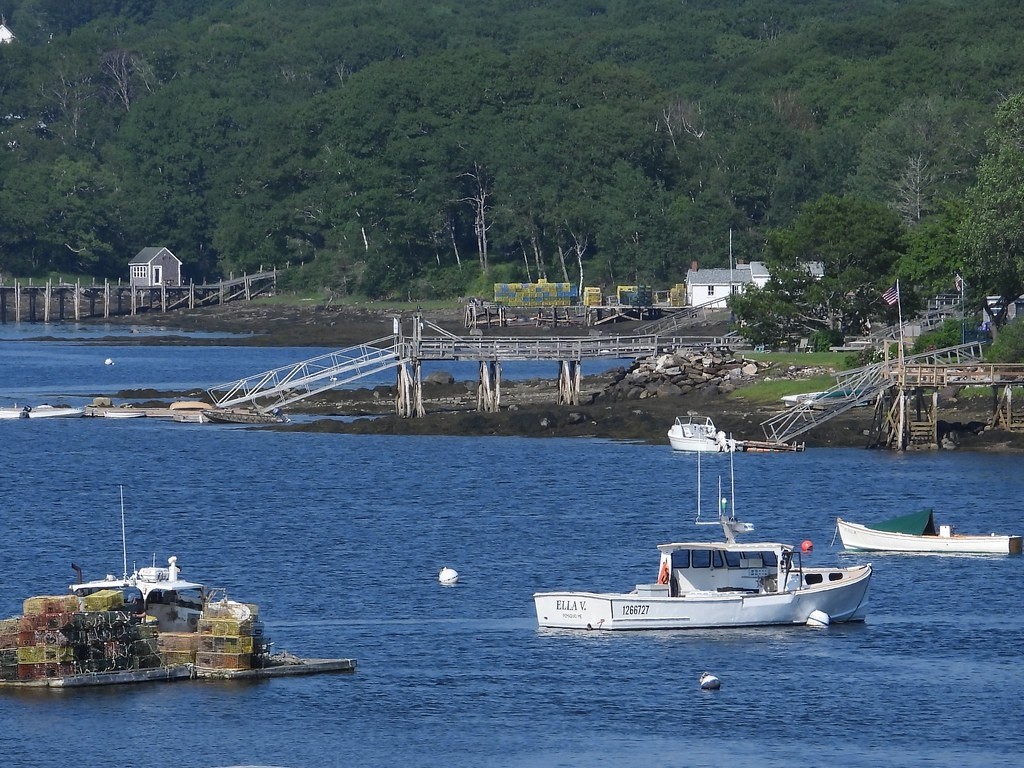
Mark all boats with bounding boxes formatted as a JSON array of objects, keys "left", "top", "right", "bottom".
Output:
[
  {"left": 666, "top": 411, "right": 728, "bottom": 452},
  {"left": 831, "top": 508, "right": 1023, "bottom": 558},
  {"left": 201, "top": 409, "right": 292, "bottom": 424},
  {"left": 532, "top": 431, "right": 874, "bottom": 632},
  {"left": 0, "top": 484, "right": 361, "bottom": 692},
  {"left": 103, "top": 410, "right": 147, "bottom": 418},
  {"left": 0, "top": 402, "right": 85, "bottom": 420}
]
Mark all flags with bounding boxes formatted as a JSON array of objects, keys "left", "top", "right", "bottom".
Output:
[
  {"left": 882, "top": 280, "right": 899, "bottom": 306},
  {"left": 954, "top": 274, "right": 967, "bottom": 292}
]
